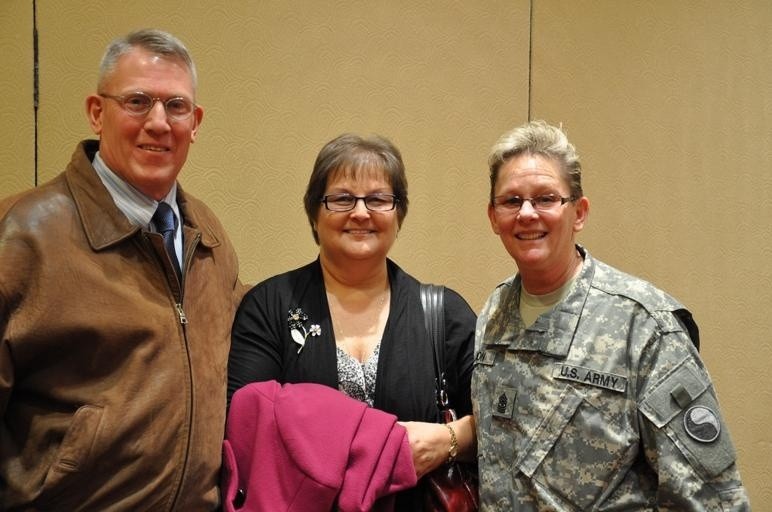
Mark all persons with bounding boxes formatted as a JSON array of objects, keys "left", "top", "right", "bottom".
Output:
[
  {"left": 225, "top": 121, "right": 479, "bottom": 512},
  {"left": 468, "top": 121, "right": 752, "bottom": 512},
  {"left": 1, "top": 27, "right": 255, "bottom": 512}
]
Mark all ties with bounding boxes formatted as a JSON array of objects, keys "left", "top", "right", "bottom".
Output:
[{"left": 150, "top": 202, "right": 184, "bottom": 298}]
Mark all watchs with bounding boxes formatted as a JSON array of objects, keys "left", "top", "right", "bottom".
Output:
[{"left": 446, "top": 422, "right": 458, "bottom": 464}]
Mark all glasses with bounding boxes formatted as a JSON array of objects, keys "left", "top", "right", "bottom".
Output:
[
  {"left": 98, "top": 91, "right": 199, "bottom": 119},
  {"left": 320, "top": 191, "right": 398, "bottom": 212},
  {"left": 494, "top": 193, "right": 576, "bottom": 214}
]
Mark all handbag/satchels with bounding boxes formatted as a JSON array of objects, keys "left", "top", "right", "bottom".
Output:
[{"left": 421, "top": 410, "right": 479, "bottom": 512}]
